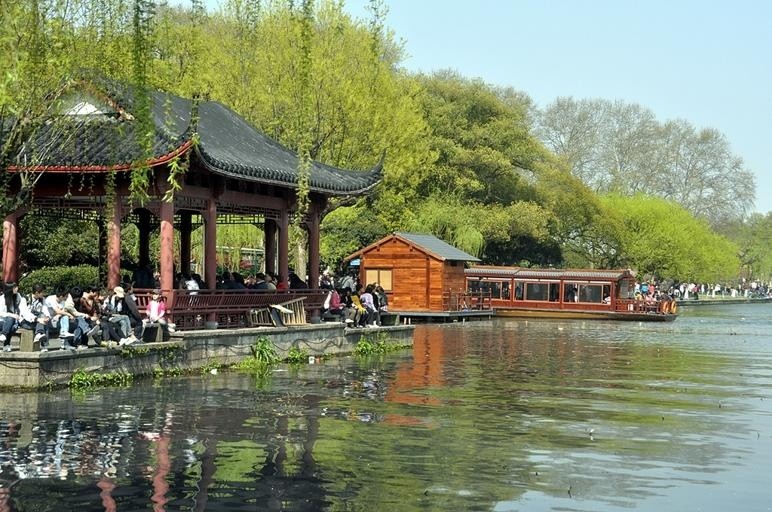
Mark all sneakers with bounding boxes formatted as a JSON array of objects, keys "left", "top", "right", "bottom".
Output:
[
  {"left": 165, "top": 327, "right": 175, "bottom": 333},
  {"left": 59, "top": 331, "right": 89, "bottom": 350},
  {"left": 355, "top": 323, "right": 363, "bottom": 328},
  {"left": 101, "top": 336, "right": 146, "bottom": 348},
  {"left": 345, "top": 319, "right": 354, "bottom": 323},
  {"left": 0, "top": 334, "right": 13, "bottom": 352},
  {"left": 33, "top": 333, "right": 49, "bottom": 352},
  {"left": 87, "top": 325, "right": 101, "bottom": 337}
]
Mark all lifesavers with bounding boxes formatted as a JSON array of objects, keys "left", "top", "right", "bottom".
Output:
[
  {"left": 670, "top": 301, "right": 678, "bottom": 314},
  {"left": 662, "top": 302, "right": 669, "bottom": 314}
]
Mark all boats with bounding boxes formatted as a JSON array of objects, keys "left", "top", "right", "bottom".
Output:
[{"left": 461, "top": 264, "right": 680, "bottom": 323}]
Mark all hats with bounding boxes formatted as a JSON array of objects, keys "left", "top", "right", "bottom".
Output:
[
  {"left": 114, "top": 286, "right": 124, "bottom": 298},
  {"left": 151, "top": 289, "right": 163, "bottom": 295}
]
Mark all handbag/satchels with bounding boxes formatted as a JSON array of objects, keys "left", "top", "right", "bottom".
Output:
[{"left": 324, "top": 289, "right": 339, "bottom": 310}]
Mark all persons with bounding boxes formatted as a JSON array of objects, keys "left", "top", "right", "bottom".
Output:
[
  {"left": 144, "top": 288, "right": 176, "bottom": 334},
  {"left": 122, "top": 267, "right": 389, "bottom": 330},
  {"left": 276, "top": 365, "right": 393, "bottom": 422},
  {"left": 0, "top": 281, "right": 147, "bottom": 354},
  {"left": 0, "top": 409, "right": 202, "bottom": 494},
  {"left": 476, "top": 277, "right": 772, "bottom": 312}
]
